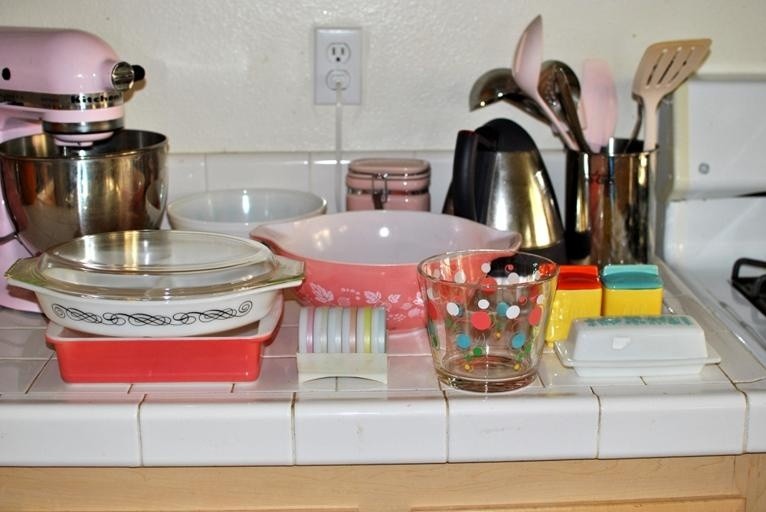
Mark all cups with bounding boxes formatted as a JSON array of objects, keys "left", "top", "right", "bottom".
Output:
[
  {"left": 417, "top": 249, "right": 560, "bottom": 393},
  {"left": 566, "top": 138, "right": 660, "bottom": 265}
]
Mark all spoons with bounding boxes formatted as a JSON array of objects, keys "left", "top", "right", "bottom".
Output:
[{"left": 470, "top": 15, "right": 620, "bottom": 153}]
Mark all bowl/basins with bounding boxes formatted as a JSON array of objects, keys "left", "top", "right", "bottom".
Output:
[
  {"left": 166, "top": 187, "right": 328, "bottom": 250},
  {"left": 251, "top": 213, "right": 523, "bottom": 335}
]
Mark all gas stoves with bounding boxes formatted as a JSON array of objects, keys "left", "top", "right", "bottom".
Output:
[{"left": 726, "top": 254, "right": 766, "bottom": 320}]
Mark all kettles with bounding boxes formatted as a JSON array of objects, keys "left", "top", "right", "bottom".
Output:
[{"left": 442, "top": 118, "right": 567, "bottom": 264}]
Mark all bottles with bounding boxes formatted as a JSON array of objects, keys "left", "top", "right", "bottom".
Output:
[{"left": 345, "top": 157, "right": 432, "bottom": 212}]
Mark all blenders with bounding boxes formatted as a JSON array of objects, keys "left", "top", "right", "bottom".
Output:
[{"left": 0, "top": 26, "right": 169, "bottom": 314}]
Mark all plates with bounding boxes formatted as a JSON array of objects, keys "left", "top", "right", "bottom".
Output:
[
  {"left": 1, "top": 230, "right": 307, "bottom": 336},
  {"left": 43, "top": 289, "right": 283, "bottom": 390}
]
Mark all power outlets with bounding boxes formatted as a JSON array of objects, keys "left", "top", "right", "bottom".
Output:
[{"left": 314, "top": 26, "right": 361, "bottom": 106}]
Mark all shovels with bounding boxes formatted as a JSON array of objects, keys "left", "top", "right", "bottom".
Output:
[{"left": 631, "top": 38, "right": 712, "bottom": 152}]
